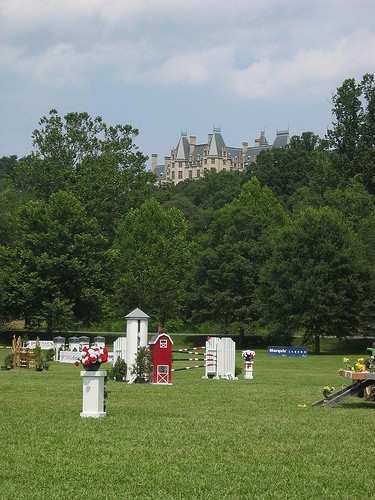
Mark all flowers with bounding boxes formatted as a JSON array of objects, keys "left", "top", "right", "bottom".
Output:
[{"left": 75, "top": 344, "right": 109, "bottom": 371}]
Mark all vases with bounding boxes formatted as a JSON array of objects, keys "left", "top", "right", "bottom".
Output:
[{"left": 79, "top": 370, "right": 106, "bottom": 418}]
